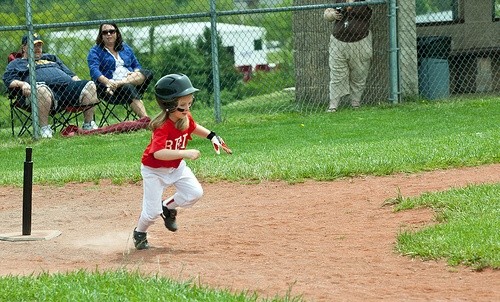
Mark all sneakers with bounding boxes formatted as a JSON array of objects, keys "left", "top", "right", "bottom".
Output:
[
  {"left": 160, "top": 200, "right": 177, "bottom": 232},
  {"left": 132, "top": 226, "right": 148, "bottom": 251}
]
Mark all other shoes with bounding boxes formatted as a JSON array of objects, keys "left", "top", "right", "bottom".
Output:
[
  {"left": 39, "top": 126, "right": 53, "bottom": 139},
  {"left": 82, "top": 120, "right": 99, "bottom": 132}
]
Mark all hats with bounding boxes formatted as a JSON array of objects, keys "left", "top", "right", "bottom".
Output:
[{"left": 21, "top": 32, "right": 44, "bottom": 46}]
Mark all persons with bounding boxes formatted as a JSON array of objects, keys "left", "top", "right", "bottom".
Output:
[
  {"left": 324, "top": 0, "right": 372, "bottom": 113},
  {"left": 133, "top": 73, "right": 232, "bottom": 250},
  {"left": 87, "top": 21, "right": 154, "bottom": 122},
  {"left": 2, "top": 32, "right": 99, "bottom": 139}
]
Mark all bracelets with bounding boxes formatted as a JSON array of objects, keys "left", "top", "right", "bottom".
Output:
[{"left": 206, "top": 130, "right": 215, "bottom": 140}]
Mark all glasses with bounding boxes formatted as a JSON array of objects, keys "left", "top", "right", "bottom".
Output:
[{"left": 101, "top": 29, "right": 117, "bottom": 35}]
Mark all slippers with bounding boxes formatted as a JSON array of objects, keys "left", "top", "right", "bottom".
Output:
[{"left": 327, "top": 108, "right": 337, "bottom": 114}]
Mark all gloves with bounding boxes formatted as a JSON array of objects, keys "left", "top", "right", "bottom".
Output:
[{"left": 206, "top": 131, "right": 232, "bottom": 155}]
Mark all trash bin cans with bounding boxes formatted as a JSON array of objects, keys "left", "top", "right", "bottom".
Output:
[{"left": 417, "top": 36, "right": 452, "bottom": 100}]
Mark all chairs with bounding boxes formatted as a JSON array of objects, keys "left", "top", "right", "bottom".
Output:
[
  {"left": 92, "top": 67, "right": 154, "bottom": 128},
  {"left": 8, "top": 52, "right": 101, "bottom": 137}
]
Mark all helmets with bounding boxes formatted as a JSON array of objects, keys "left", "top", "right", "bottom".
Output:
[{"left": 154, "top": 73, "right": 200, "bottom": 112}]
